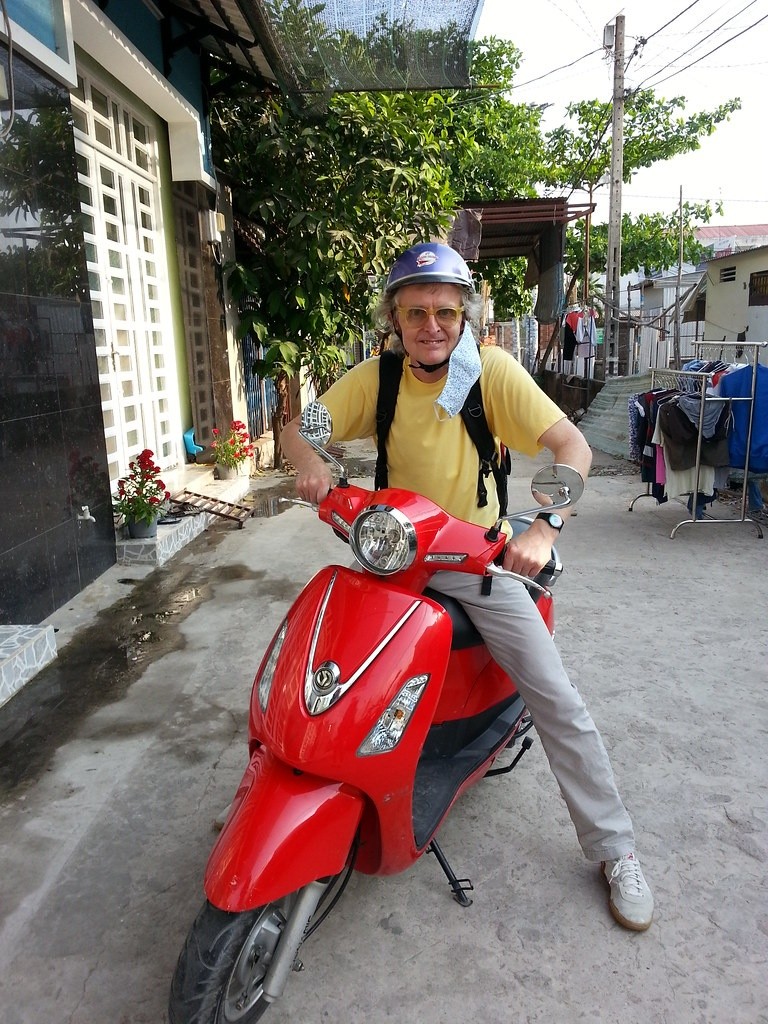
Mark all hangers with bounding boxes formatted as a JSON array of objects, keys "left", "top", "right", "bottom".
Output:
[
  {"left": 684, "top": 347, "right": 760, "bottom": 373},
  {"left": 556, "top": 301, "right": 595, "bottom": 315},
  {"left": 647, "top": 374, "right": 726, "bottom": 408}
]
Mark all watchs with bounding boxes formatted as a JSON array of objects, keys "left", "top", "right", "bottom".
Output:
[{"left": 535, "top": 511, "right": 564, "bottom": 534}]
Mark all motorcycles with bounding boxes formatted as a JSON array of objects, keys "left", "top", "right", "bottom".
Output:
[{"left": 165, "top": 401, "right": 586, "bottom": 1017}]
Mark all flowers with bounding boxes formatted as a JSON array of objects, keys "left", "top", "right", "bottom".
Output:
[
  {"left": 210, "top": 421, "right": 255, "bottom": 468},
  {"left": 112, "top": 449, "right": 170, "bottom": 528}
]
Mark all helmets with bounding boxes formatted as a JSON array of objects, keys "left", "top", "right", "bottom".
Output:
[{"left": 384, "top": 243, "right": 476, "bottom": 295}]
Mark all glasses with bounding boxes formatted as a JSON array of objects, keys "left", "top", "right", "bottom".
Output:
[{"left": 395, "top": 305, "right": 466, "bottom": 328}]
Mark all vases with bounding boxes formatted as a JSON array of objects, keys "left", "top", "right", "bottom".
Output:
[
  {"left": 216, "top": 462, "right": 236, "bottom": 480},
  {"left": 125, "top": 514, "right": 158, "bottom": 539}
]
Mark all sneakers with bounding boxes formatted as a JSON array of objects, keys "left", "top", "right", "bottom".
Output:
[
  {"left": 215, "top": 803, "right": 231, "bottom": 830},
  {"left": 601, "top": 852, "right": 655, "bottom": 930}
]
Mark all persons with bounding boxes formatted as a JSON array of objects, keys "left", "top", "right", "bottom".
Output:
[{"left": 276, "top": 240, "right": 658, "bottom": 932}]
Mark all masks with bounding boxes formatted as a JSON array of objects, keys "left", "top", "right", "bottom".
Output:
[{"left": 433, "top": 319, "right": 482, "bottom": 421}]
